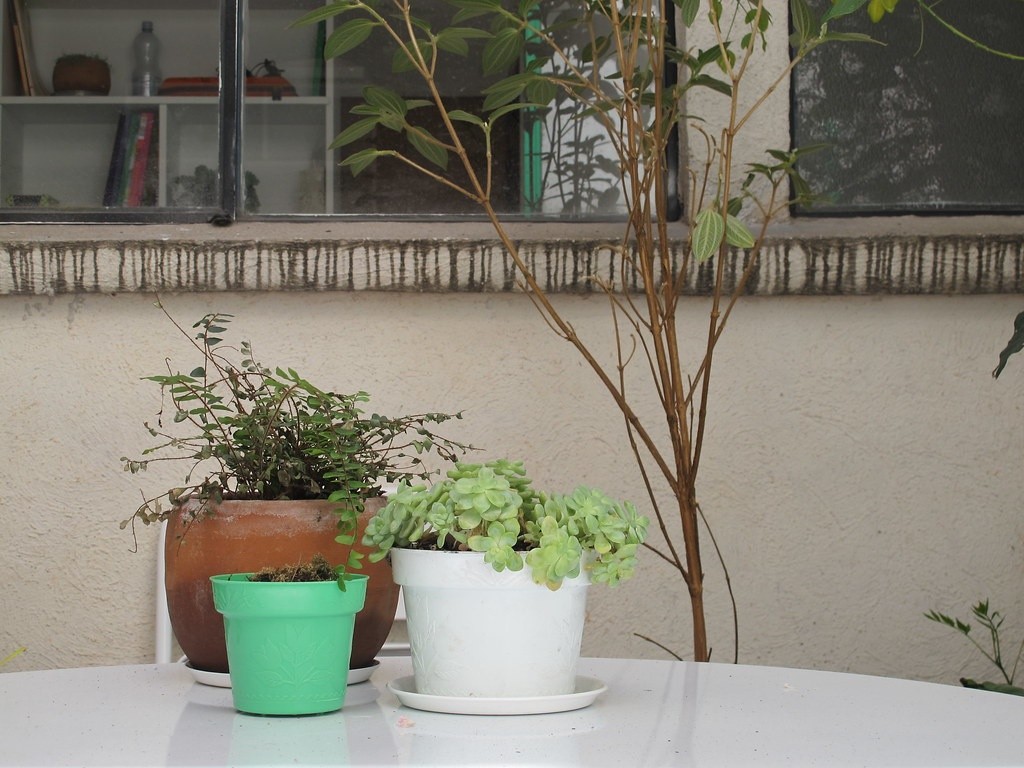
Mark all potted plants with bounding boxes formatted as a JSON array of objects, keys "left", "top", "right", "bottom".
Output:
[
  {"left": 360, "top": 458, "right": 649, "bottom": 697},
  {"left": 120, "top": 284, "right": 486, "bottom": 674},
  {"left": 210, "top": 552, "right": 368, "bottom": 716}
]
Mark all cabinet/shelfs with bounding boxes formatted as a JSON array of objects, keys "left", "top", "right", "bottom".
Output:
[{"left": 0, "top": 0, "right": 336, "bottom": 220}]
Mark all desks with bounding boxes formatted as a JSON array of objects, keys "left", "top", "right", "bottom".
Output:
[{"left": 0, "top": 656, "right": 1024, "bottom": 768}]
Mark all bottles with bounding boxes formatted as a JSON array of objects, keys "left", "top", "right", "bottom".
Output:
[{"left": 134, "top": 21, "right": 159, "bottom": 97}]
